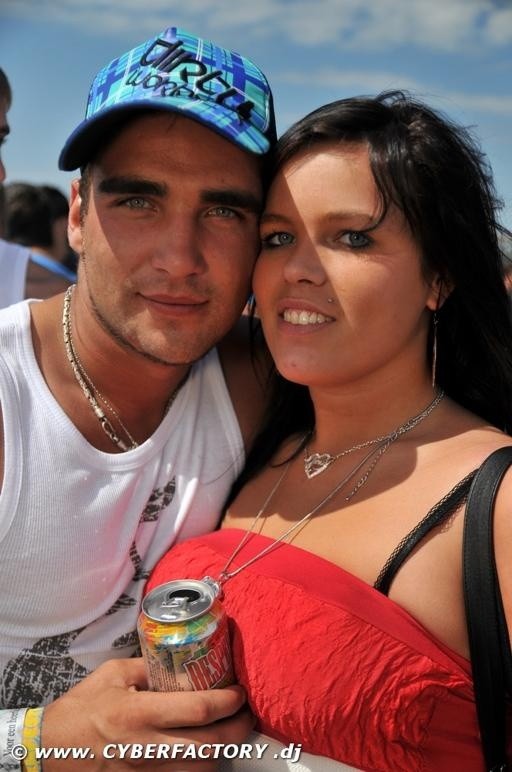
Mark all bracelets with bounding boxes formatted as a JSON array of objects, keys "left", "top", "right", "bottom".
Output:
[{"left": 1, "top": 708, "right": 44, "bottom": 769}]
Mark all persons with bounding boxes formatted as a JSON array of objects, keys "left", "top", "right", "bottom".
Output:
[
  {"left": 137, "top": 87, "right": 511, "bottom": 772},
  {"left": 1, "top": 70, "right": 68, "bottom": 313},
  {"left": 41, "top": 186, "right": 82, "bottom": 279},
  {"left": 0, "top": 27, "right": 272, "bottom": 770},
  {"left": 236, "top": 296, "right": 264, "bottom": 326},
  {"left": 0, "top": 182, "right": 52, "bottom": 261},
  {"left": 499, "top": 259, "right": 511, "bottom": 292}
]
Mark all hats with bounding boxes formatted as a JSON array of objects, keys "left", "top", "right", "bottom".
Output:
[{"left": 59, "top": 28, "right": 277, "bottom": 172}]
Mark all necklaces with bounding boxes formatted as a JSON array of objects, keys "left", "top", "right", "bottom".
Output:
[
  {"left": 207, "top": 389, "right": 445, "bottom": 608},
  {"left": 58, "top": 279, "right": 210, "bottom": 456}
]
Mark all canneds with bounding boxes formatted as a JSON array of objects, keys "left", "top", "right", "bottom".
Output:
[{"left": 136, "top": 578, "right": 234, "bottom": 691}]
